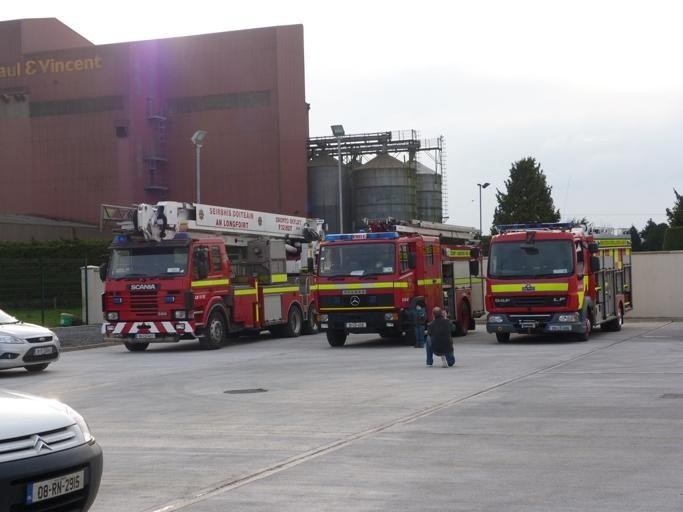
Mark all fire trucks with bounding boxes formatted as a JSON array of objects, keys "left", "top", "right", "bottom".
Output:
[
  {"left": 307, "top": 217, "right": 485, "bottom": 347},
  {"left": 469, "top": 222, "right": 633, "bottom": 344},
  {"left": 97, "top": 200, "right": 331, "bottom": 351}
]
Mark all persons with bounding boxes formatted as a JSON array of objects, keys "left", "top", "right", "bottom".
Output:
[{"left": 423, "top": 306, "right": 457, "bottom": 369}]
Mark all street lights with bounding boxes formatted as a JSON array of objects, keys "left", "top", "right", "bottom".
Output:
[
  {"left": 330, "top": 125, "right": 344, "bottom": 234},
  {"left": 189, "top": 129, "right": 207, "bottom": 204},
  {"left": 476, "top": 181, "right": 490, "bottom": 237}
]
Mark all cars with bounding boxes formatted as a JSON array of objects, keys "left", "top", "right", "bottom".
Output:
[
  {"left": 0, "top": 309, "right": 60, "bottom": 371},
  {"left": 0, "top": 387, "right": 102, "bottom": 511}
]
